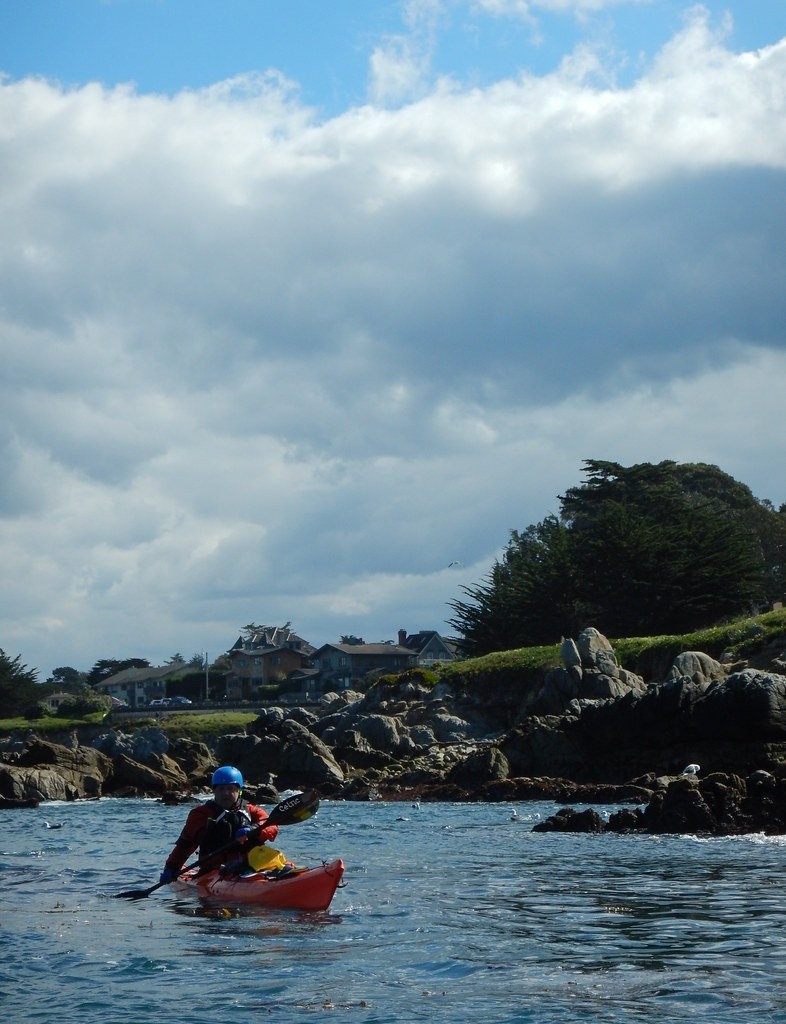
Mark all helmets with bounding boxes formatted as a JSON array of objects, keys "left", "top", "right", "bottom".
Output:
[{"left": 211, "top": 765, "right": 243, "bottom": 790}]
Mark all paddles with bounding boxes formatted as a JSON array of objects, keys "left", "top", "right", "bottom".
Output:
[{"left": 111, "top": 789, "right": 321, "bottom": 901}]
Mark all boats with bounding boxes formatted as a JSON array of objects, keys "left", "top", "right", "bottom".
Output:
[{"left": 170, "top": 857, "right": 344, "bottom": 911}]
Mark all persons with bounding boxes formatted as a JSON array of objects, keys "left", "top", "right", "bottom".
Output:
[{"left": 160, "top": 766, "right": 278, "bottom": 885}]
